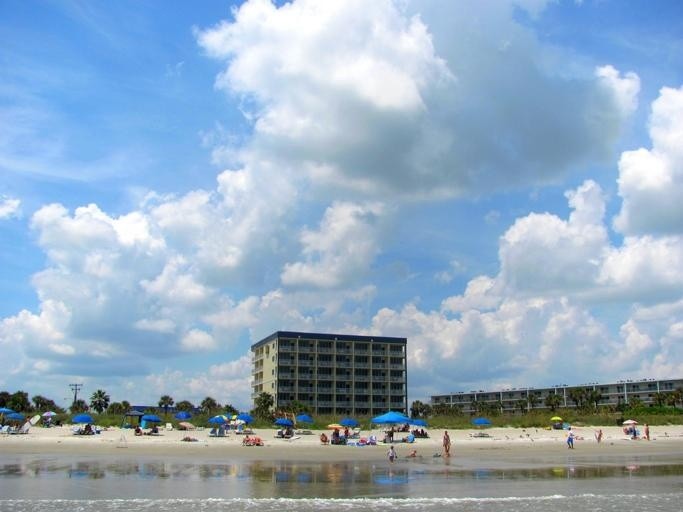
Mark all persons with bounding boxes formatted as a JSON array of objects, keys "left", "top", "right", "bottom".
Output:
[{"left": 78, "top": 421, "right": 654, "bottom": 464}]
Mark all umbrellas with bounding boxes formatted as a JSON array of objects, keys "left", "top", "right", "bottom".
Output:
[{"left": 622, "top": 419, "right": 639, "bottom": 426}]
